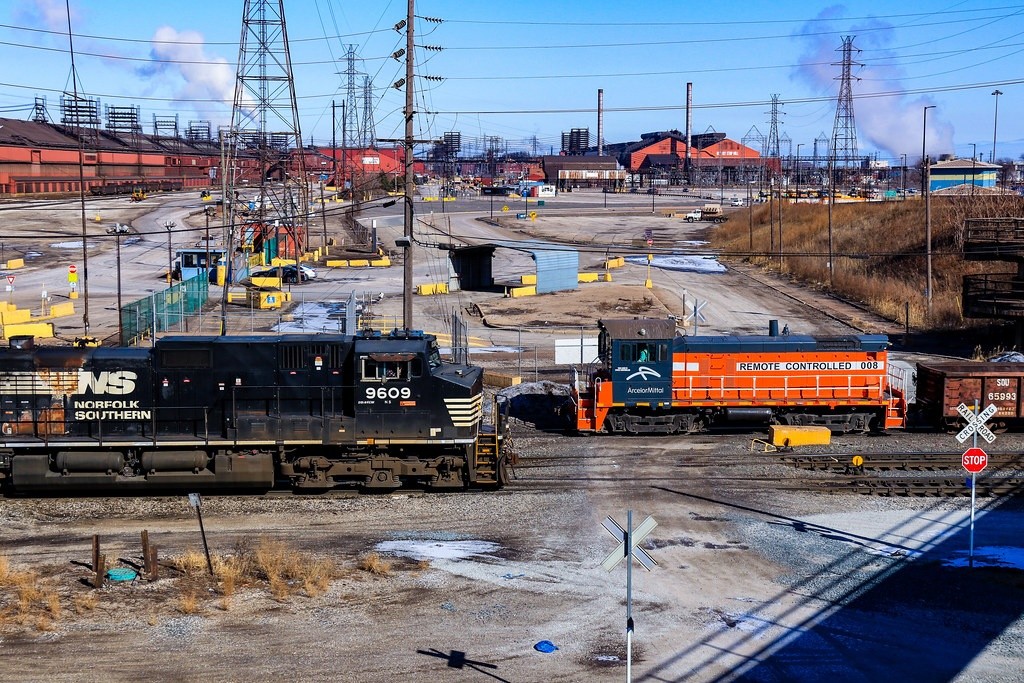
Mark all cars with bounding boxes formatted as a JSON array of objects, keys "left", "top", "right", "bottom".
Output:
[
  {"left": 683, "top": 189, "right": 689, "bottom": 192},
  {"left": 896, "top": 188, "right": 906, "bottom": 193},
  {"left": 284, "top": 264, "right": 317, "bottom": 280},
  {"left": 252, "top": 267, "right": 307, "bottom": 283},
  {"left": 630, "top": 188, "right": 638, "bottom": 193},
  {"left": 908, "top": 188, "right": 917, "bottom": 193},
  {"left": 647, "top": 188, "right": 658, "bottom": 194}
]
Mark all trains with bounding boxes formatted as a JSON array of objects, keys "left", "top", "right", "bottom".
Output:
[
  {"left": 565, "top": 317, "right": 1024, "bottom": 436},
  {"left": 0, "top": 327, "right": 518, "bottom": 494}
]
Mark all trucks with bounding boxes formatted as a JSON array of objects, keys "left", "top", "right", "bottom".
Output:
[
  {"left": 685, "top": 208, "right": 728, "bottom": 223},
  {"left": 760, "top": 188, "right": 842, "bottom": 197}
]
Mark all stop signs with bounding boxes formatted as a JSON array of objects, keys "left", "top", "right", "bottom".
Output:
[
  {"left": 68, "top": 265, "right": 77, "bottom": 273},
  {"left": 962, "top": 448, "right": 988, "bottom": 474}
]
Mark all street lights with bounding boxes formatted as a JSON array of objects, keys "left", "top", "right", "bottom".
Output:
[{"left": 921, "top": 106, "right": 936, "bottom": 197}]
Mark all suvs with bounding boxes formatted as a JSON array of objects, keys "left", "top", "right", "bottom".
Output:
[{"left": 731, "top": 199, "right": 743, "bottom": 207}]
